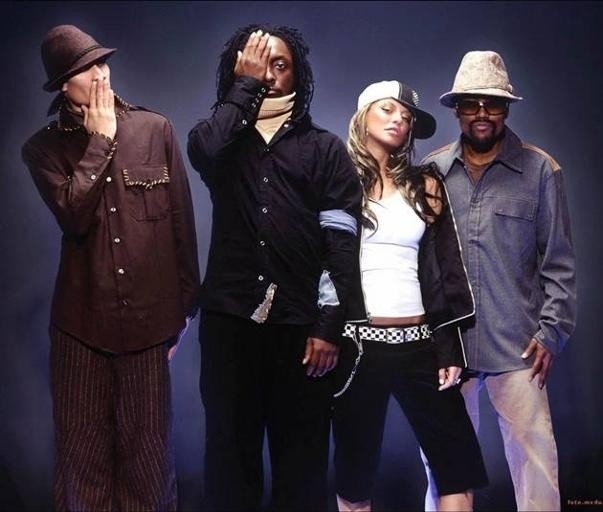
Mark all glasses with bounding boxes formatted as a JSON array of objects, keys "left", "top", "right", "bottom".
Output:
[{"left": 452, "top": 97, "right": 511, "bottom": 116}]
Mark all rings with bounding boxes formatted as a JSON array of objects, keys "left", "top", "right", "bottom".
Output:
[{"left": 455, "top": 378, "right": 461, "bottom": 383}]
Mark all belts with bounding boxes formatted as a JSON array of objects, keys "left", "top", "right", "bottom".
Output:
[{"left": 341, "top": 322, "right": 432, "bottom": 345}]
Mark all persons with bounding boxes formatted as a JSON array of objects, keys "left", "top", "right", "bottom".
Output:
[
  {"left": 188, "top": 25, "right": 363, "bottom": 512},
  {"left": 331, "top": 80, "right": 489, "bottom": 512},
  {"left": 420, "top": 51, "right": 578, "bottom": 512},
  {"left": 20, "top": 25, "right": 202, "bottom": 512}
]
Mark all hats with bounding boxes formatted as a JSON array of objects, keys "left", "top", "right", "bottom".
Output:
[
  {"left": 357, "top": 80, "right": 436, "bottom": 139},
  {"left": 439, "top": 50, "right": 524, "bottom": 108},
  {"left": 41, "top": 24, "right": 117, "bottom": 92}
]
